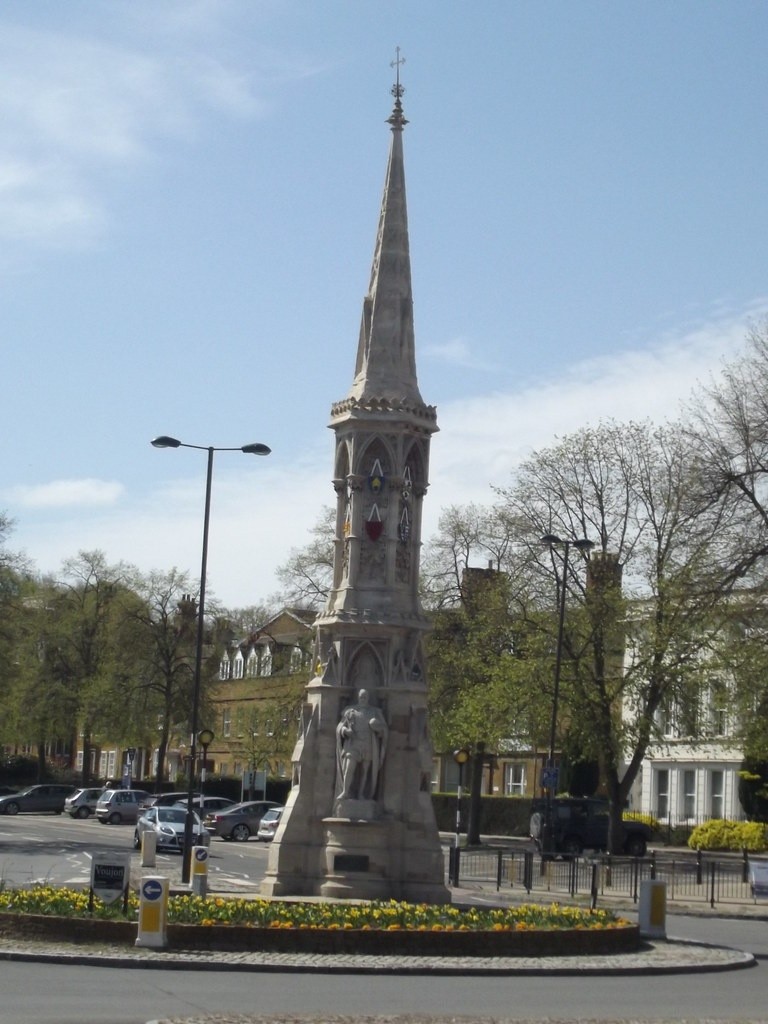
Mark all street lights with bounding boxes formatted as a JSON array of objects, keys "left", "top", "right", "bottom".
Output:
[
  {"left": 539, "top": 531, "right": 596, "bottom": 877},
  {"left": 150, "top": 436, "right": 271, "bottom": 885}
]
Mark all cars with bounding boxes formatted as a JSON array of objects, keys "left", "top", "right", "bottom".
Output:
[
  {"left": 133, "top": 806, "right": 211, "bottom": 852},
  {"left": 0, "top": 784, "right": 76, "bottom": 815},
  {"left": 172, "top": 797, "right": 237, "bottom": 820},
  {"left": 257, "top": 806, "right": 285, "bottom": 841},
  {"left": 203, "top": 800, "right": 286, "bottom": 843},
  {"left": 137, "top": 792, "right": 208, "bottom": 821},
  {"left": 94, "top": 789, "right": 151, "bottom": 825},
  {"left": 63, "top": 787, "right": 109, "bottom": 819}
]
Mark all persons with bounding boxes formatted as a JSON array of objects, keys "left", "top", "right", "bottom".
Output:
[
  {"left": 336, "top": 688, "right": 389, "bottom": 800},
  {"left": 102, "top": 781, "right": 112, "bottom": 790}
]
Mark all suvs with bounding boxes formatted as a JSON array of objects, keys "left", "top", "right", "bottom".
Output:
[{"left": 529, "top": 800, "right": 650, "bottom": 861}]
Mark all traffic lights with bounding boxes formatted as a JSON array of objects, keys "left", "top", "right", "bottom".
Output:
[
  {"left": 453, "top": 747, "right": 471, "bottom": 889},
  {"left": 197, "top": 729, "right": 214, "bottom": 845}
]
